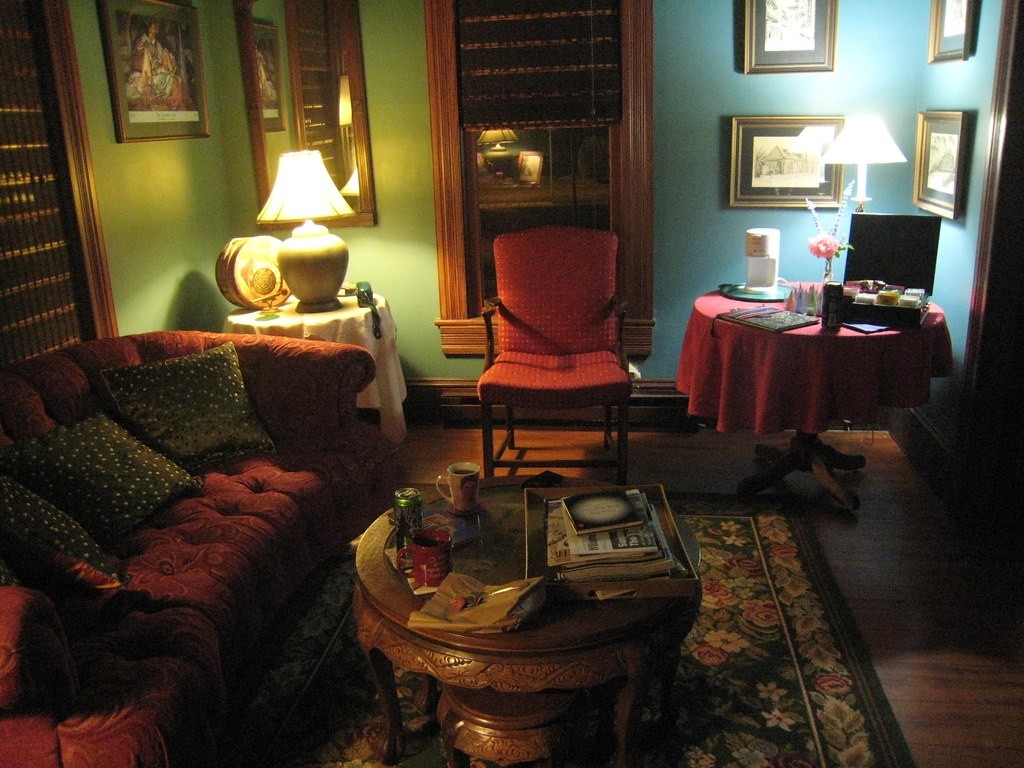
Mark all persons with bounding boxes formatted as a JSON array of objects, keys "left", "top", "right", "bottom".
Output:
[{"left": 458, "top": 477, "right": 479, "bottom": 503}]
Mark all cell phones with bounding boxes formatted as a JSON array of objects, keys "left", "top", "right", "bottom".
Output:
[{"left": 520, "top": 470, "right": 562, "bottom": 491}]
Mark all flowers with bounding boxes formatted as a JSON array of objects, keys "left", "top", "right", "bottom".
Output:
[{"left": 805, "top": 180, "right": 855, "bottom": 279}]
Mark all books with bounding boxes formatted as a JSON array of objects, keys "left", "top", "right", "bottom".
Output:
[
  {"left": 524, "top": 483, "right": 700, "bottom": 600},
  {"left": 717, "top": 309, "right": 821, "bottom": 333}
]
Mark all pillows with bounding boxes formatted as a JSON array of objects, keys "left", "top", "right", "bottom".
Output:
[
  {"left": 0, "top": 471, "right": 132, "bottom": 589},
  {"left": 98, "top": 340, "right": 274, "bottom": 474},
  {"left": 1, "top": 404, "right": 201, "bottom": 558}
]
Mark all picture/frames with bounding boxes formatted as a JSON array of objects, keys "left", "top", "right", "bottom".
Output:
[
  {"left": 727, "top": 115, "right": 843, "bottom": 210},
  {"left": 928, "top": 0, "right": 973, "bottom": 65},
  {"left": 96, "top": 0, "right": 211, "bottom": 143},
  {"left": 913, "top": 111, "right": 968, "bottom": 220},
  {"left": 517, "top": 151, "right": 543, "bottom": 182},
  {"left": 734, "top": 0, "right": 839, "bottom": 74},
  {"left": 249, "top": 22, "right": 285, "bottom": 132}
]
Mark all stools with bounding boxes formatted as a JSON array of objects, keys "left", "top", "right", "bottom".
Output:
[{"left": 437, "top": 682, "right": 575, "bottom": 768}]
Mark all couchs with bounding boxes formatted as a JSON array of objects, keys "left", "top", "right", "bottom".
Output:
[{"left": 0, "top": 331, "right": 397, "bottom": 768}]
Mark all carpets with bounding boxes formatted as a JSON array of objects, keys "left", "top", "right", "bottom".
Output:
[{"left": 191, "top": 484, "right": 915, "bottom": 768}]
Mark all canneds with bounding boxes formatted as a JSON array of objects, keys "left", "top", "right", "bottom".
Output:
[{"left": 392, "top": 487, "right": 424, "bottom": 559}]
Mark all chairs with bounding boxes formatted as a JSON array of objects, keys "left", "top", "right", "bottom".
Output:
[{"left": 478, "top": 226, "right": 632, "bottom": 485}]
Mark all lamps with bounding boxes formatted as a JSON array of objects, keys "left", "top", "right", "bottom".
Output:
[
  {"left": 340, "top": 166, "right": 360, "bottom": 196},
  {"left": 821, "top": 114, "right": 907, "bottom": 213},
  {"left": 476, "top": 129, "right": 519, "bottom": 151},
  {"left": 256, "top": 149, "right": 356, "bottom": 312},
  {"left": 792, "top": 124, "right": 836, "bottom": 153},
  {"left": 338, "top": 75, "right": 354, "bottom": 126}
]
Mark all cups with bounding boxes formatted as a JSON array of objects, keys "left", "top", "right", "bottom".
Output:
[
  {"left": 395, "top": 528, "right": 453, "bottom": 587},
  {"left": 435, "top": 461, "right": 481, "bottom": 511}
]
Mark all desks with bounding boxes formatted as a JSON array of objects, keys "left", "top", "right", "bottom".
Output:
[
  {"left": 674, "top": 282, "right": 953, "bottom": 510},
  {"left": 224, "top": 292, "right": 406, "bottom": 444}
]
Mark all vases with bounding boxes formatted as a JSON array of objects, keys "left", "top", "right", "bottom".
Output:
[{"left": 822, "top": 272, "right": 833, "bottom": 286}]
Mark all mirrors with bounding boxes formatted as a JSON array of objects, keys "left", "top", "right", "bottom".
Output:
[{"left": 235, "top": 0, "right": 376, "bottom": 233}]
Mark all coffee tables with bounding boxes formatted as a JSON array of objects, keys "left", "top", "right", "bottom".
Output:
[{"left": 349, "top": 475, "right": 700, "bottom": 768}]
